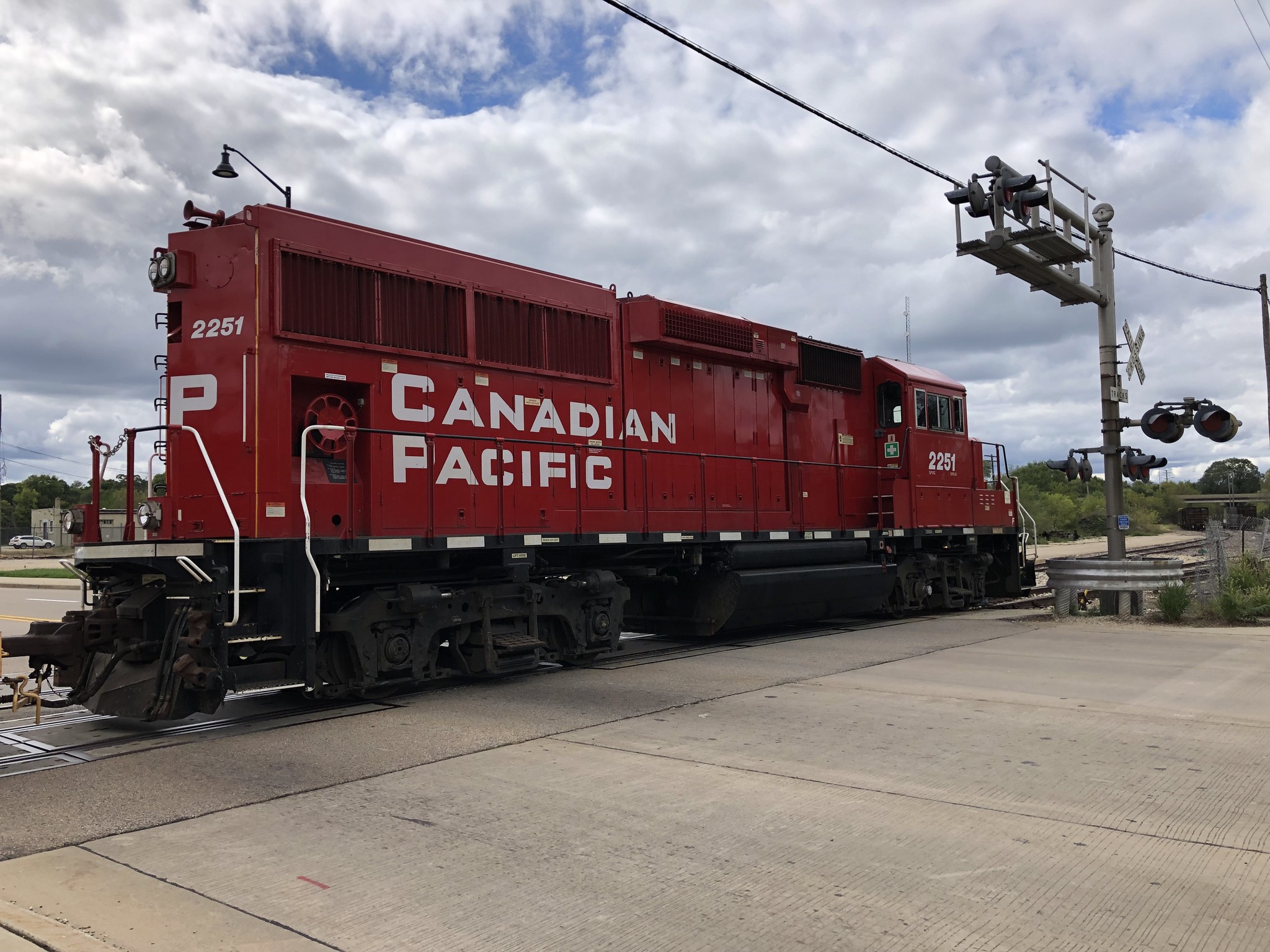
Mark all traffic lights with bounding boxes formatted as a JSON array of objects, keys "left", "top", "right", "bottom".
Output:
[
  {"left": 1120, "top": 447, "right": 1168, "bottom": 484},
  {"left": 944, "top": 179, "right": 994, "bottom": 229},
  {"left": 993, "top": 170, "right": 1048, "bottom": 225},
  {"left": 1046, "top": 456, "right": 1093, "bottom": 483},
  {"left": 1140, "top": 400, "right": 1230, "bottom": 441}
]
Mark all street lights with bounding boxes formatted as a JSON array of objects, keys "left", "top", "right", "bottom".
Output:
[{"left": 213, "top": 144, "right": 291, "bottom": 210}]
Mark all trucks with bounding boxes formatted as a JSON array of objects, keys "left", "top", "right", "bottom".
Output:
[{"left": 1222, "top": 506, "right": 1258, "bottom": 529}]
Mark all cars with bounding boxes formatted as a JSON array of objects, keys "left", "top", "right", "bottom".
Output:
[{"left": 9, "top": 535, "right": 56, "bottom": 549}]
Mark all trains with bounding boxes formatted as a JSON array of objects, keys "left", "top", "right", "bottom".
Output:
[
  {"left": 1177, "top": 507, "right": 1208, "bottom": 530},
  {"left": 1, "top": 198, "right": 1039, "bottom": 726}
]
